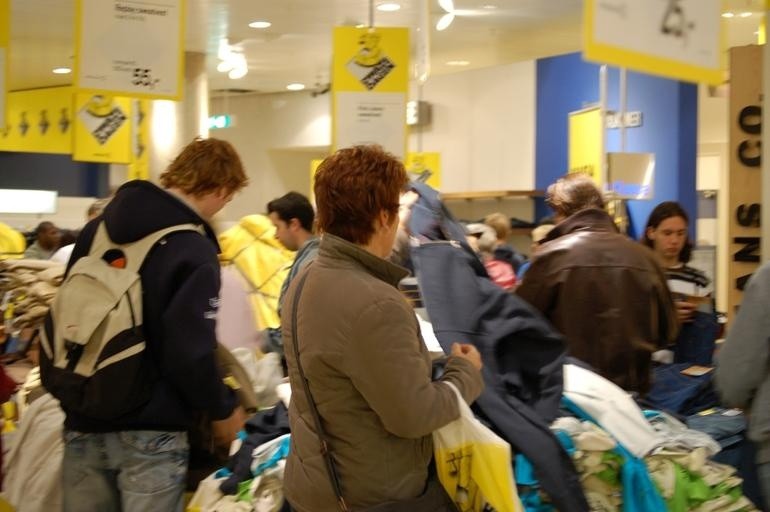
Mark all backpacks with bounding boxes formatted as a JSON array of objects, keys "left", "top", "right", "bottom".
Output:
[{"left": 37, "top": 225, "right": 208, "bottom": 406}]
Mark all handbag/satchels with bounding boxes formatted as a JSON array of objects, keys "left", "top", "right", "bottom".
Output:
[{"left": 347, "top": 467, "right": 459, "bottom": 511}]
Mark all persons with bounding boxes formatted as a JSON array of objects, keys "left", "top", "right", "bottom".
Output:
[
  {"left": 280, "top": 143, "right": 485, "bottom": 511},
  {"left": 60, "top": 138, "right": 252, "bottom": 512},
  {"left": 1, "top": 172, "right": 770, "bottom": 511}
]
